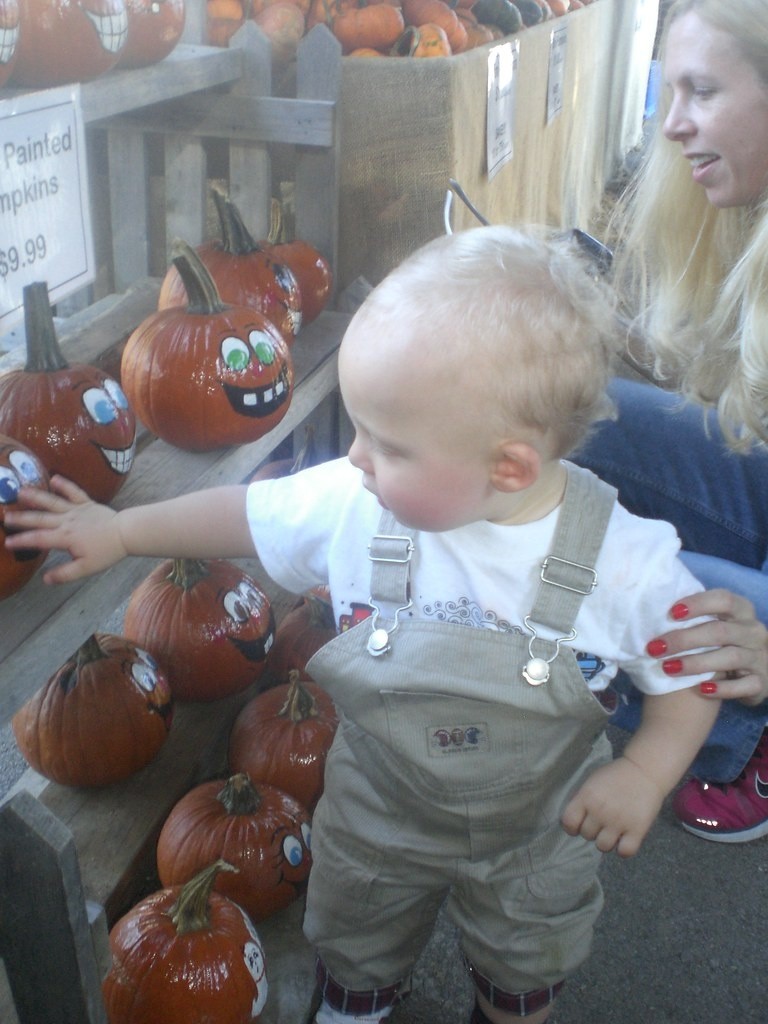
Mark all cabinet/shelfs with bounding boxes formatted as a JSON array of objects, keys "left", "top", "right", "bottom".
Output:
[{"left": 1, "top": 270, "right": 398, "bottom": 1020}]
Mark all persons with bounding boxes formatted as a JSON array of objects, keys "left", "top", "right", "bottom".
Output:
[
  {"left": 549, "top": 1, "right": 767, "bottom": 843},
  {"left": 0, "top": 226, "right": 731, "bottom": 1024}
]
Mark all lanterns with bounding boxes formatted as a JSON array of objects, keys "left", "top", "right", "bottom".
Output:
[
  {"left": 230, "top": 668, "right": 342, "bottom": 813},
  {"left": 120, "top": 235, "right": 296, "bottom": 452},
  {"left": 112, "top": 1, "right": 186, "bottom": 71},
  {"left": 258, "top": 187, "right": 332, "bottom": 327},
  {"left": 123, "top": 550, "right": 276, "bottom": 704},
  {"left": 0, "top": 432, "right": 56, "bottom": 604},
  {"left": 158, "top": 771, "right": 313, "bottom": 924},
  {"left": 22, "top": 0, "right": 129, "bottom": 86},
  {"left": 0, "top": 281, "right": 136, "bottom": 512},
  {"left": 102, "top": 858, "right": 269, "bottom": 1022},
  {"left": 1, "top": 0, "right": 25, "bottom": 93},
  {"left": 158, "top": 185, "right": 303, "bottom": 356},
  {"left": 11, "top": 633, "right": 174, "bottom": 788}
]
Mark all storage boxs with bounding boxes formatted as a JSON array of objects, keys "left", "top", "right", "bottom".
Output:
[{"left": 139, "top": 0, "right": 666, "bottom": 312}]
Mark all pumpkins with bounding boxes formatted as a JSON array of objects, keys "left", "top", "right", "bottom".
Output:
[
  {"left": 0, "top": 0, "right": 337, "bottom": 1024},
  {"left": 209, "top": 0, "right": 580, "bottom": 58}
]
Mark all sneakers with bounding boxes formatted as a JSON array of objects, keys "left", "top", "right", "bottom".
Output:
[{"left": 673, "top": 716, "right": 768, "bottom": 844}]
